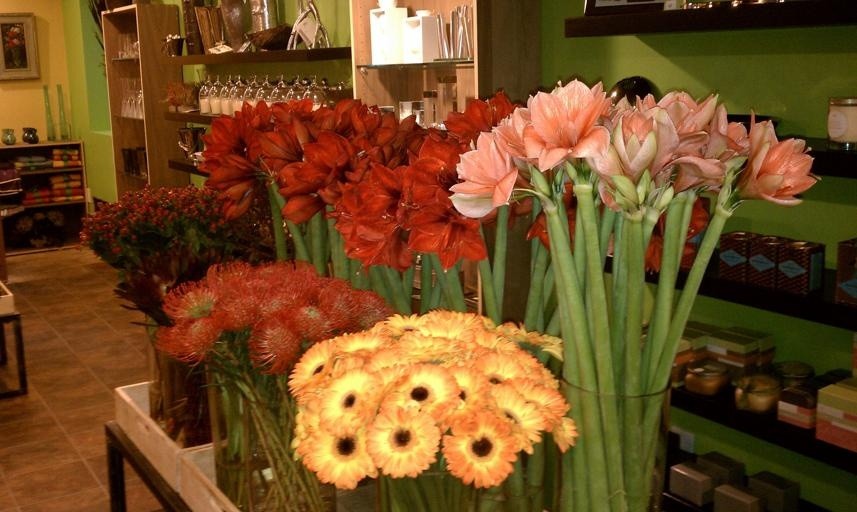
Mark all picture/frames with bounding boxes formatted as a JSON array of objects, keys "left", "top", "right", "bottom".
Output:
[{"left": 0, "top": 12, "right": 41, "bottom": 82}]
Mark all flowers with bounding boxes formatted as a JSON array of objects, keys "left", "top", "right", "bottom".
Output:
[
  {"left": 153, "top": 259, "right": 398, "bottom": 512},
  {"left": 447, "top": 80, "right": 819, "bottom": 512},
  {"left": 197, "top": 87, "right": 522, "bottom": 322},
  {"left": 78, "top": 183, "right": 305, "bottom": 450},
  {"left": 286, "top": 310, "right": 580, "bottom": 512}
]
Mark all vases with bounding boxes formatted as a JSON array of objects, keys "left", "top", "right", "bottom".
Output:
[
  {"left": 387, "top": 471, "right": 479, "bottom": 512},
  {"left": 214, "top": 398, "right": 335, "bottom": 512},
  {"left": 145, "top": 334, "right": 226, "bottom": 448},
  {"left": 559, "top": 379, "right": 672, "bottom": 512}
]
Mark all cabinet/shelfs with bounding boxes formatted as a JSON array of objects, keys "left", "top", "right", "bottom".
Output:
[
  {"left": 1, "top": 141, "right": 88, "bottom": 257},
  {"left": 100, "top": 4, "right": 190, "bottom": 203},
  {"left": 605, "top": 139, "right": 857, "bottom": 512},
  {"left": 349, "top": 0, "right": 543, "bottom": 129},
  {"left": 564, "top": 0, "right": 857, "bottom": 38},
  {"left": 166, "top": 48, "right": 349, "bottom": 178}
]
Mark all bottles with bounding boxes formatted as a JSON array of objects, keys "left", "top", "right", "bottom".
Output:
[{"left": 827, "top": 97, "right": 857, "bottom": 151}]
